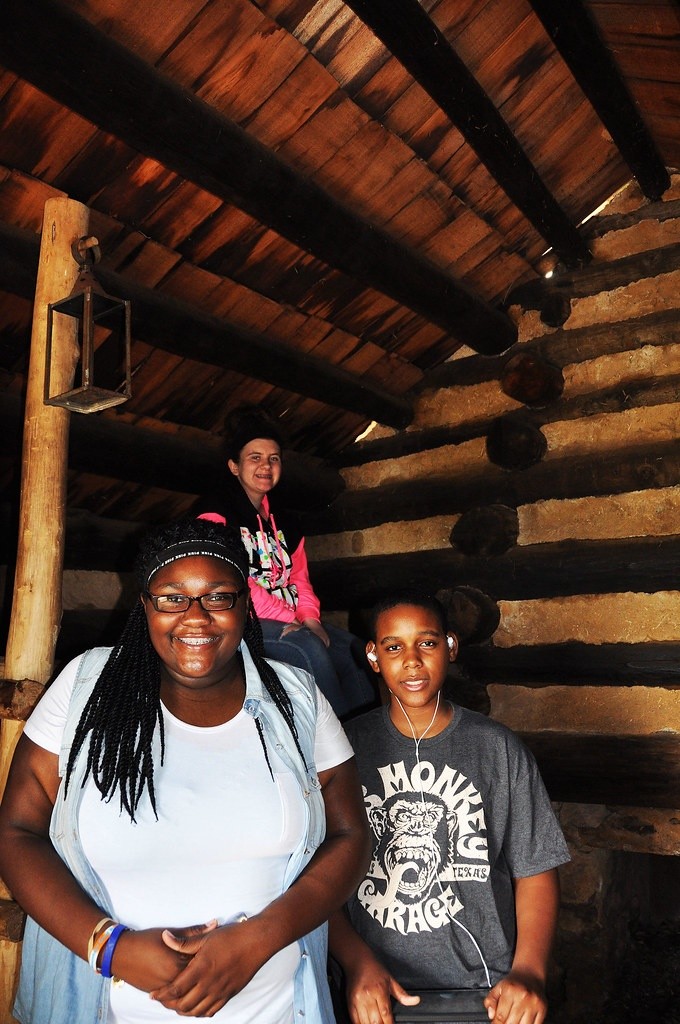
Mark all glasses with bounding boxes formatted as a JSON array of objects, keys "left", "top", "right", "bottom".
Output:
[{"left": 145, "top": 587, "right": 244, "bottom": 613}]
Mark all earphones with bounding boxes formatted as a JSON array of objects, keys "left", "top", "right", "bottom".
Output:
[
  {"left": 367, "top": 653, "right": 378, "bottom": 662},
  {"left": 448, "top": 637, "right": 454, "bottom": 649}
]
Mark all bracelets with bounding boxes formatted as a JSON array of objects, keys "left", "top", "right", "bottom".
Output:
[{"left": 88, "top": 918, "right": 127, "bottom": 978}]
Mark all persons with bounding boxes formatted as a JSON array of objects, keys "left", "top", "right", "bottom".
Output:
[
  {"left": 187, "top": 418, "right": 384, "bottom": 723},
  {"left": 328, "top": 588, "right": 572, "bottom": 1024},
  {"left": 0, "top": 517, "right": 373, "bottom": 1024}
]
